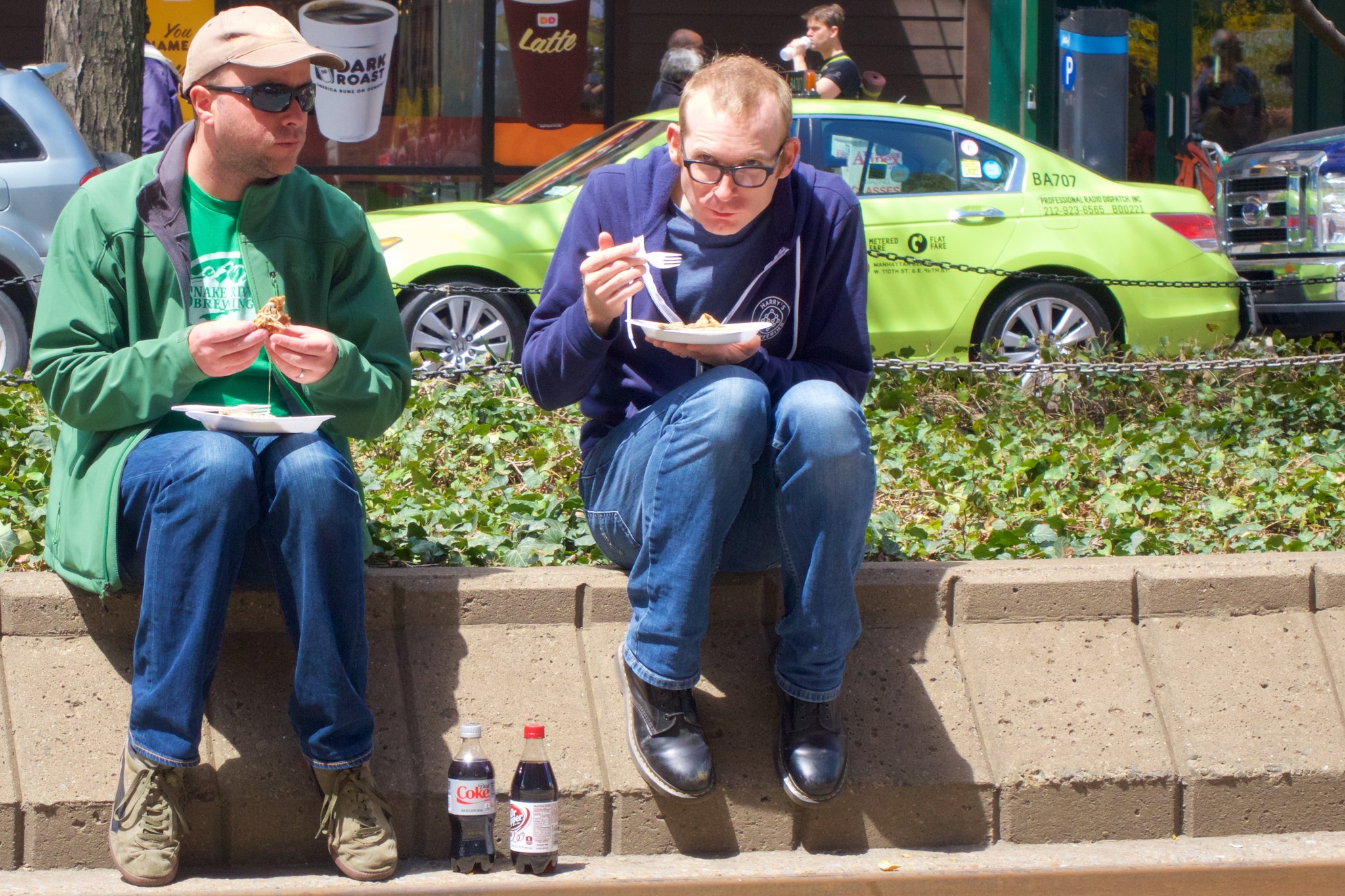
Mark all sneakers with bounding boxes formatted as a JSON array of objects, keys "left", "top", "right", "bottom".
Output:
[
  {"left": 106, "top": 733, "right": 195, "bottom": 887},
  {"left": 305, "top": 756, "right": 398, "bottom": 881}
]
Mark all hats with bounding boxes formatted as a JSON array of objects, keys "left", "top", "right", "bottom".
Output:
[{"left": 177, "top": 5, "right": 347, "bottom": 103}]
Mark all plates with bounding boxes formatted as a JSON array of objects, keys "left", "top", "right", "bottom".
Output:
[
  {"left": 185, "top": 412, "right": 336, "bottom": 434},
  {"left": 625, "top": 319, "right": 772, "bottom": 345}
]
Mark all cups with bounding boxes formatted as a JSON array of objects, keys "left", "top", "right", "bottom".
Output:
[
  {"left": 503, "top": 0, "right": 591, "bottom": 130},
  {"left": 779, "top": 36, "right": 811, "bottom": 61},
  {"left": 298, "top": 0, "right": 398, "bottom": 142}
]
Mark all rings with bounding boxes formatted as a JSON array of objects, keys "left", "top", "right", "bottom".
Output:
[{"left": 295, "top": 368, "right": 306, "bottom": 380}]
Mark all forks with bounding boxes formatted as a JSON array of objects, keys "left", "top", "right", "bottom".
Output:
[
  {"left": 586, "top": 251, "right": 682, "bottom": 269},
  {"left": 171, "top": 404, "right": 271, "bottom": 414}
]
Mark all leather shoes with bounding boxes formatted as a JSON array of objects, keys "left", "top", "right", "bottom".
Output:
[
  {"left": 773, "top": 684, "right": 850, "bottom": 807},
  {"left": 615, "top": 641, "right": 718, "bottom": 803}
]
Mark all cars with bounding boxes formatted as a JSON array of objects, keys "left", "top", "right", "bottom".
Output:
[
  {"left": 1215, "top": 123, "right": 1345, "bottom": 337},
  {"left": 0, "top": 57, "right": 107, "bottom": 385},
  {"left": 364, "top": 96, "right": 1243, "bottom": 377}
]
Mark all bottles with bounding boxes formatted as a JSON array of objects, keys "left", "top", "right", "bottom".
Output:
[
  {"left": 510, "top": 724, "right": 559, "bottom": 875},
  {"left": 448, "top": 724, "right": 496, "bottom": 874}
]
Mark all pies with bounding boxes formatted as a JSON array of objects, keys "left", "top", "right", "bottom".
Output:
[
  {"left": 254, "top": 295, "right": 290, "bottom": 336},
  {"left": 659, "top": 313, "right": 724, "bottom": 329}
]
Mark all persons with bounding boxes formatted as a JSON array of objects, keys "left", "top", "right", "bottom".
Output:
[
  {"left": 525, "top": 54, "right": 879, "bottom": 808},
  {"left": 640, "top": 3, "right": 860, "bottom": 112},
  {"left": 1134, "top": 28, "right": 1295, "bottom": 188},
  {"left": 30, "top": 7, "right": 412, "bottom": 884}
]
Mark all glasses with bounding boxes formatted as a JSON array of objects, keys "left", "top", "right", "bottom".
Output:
[
  {"left": 680, "top": 129, "right": 788, "bottom": 188},
  {"left": 201, "top": 82, "right": 318, "bottom": 114},
  {"left": 1225, "top": 41, "right": 1245, "bottom": 49}
]
